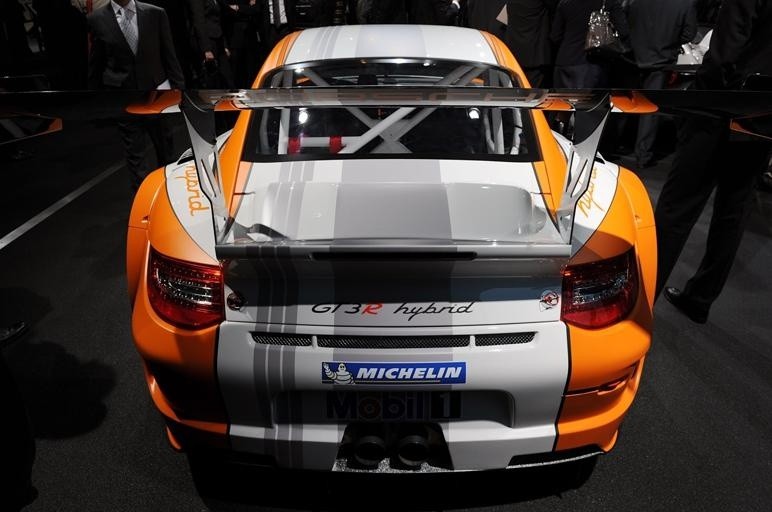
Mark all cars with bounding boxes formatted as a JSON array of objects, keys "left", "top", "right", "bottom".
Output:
[{"left": 126, "top": 24, "right": 659, "bottom": 474}]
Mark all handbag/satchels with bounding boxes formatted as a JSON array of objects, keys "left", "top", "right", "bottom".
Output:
[
  {"left": 585, "top": 10, "right": 619, "bottom": 50},
  {"left": 184, "top": 58, "right": 230, "bottom": 90}
]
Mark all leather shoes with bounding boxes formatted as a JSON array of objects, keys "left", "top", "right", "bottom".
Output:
[{"left": 663, "top": 287, "right": 709, "bottom": 324}]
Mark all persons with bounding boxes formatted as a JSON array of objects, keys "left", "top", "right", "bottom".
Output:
[
  {"left": 1, "top": 319, "right": 30, "bottom": 348},
  {"left": 549, "top": 2, "right": 770, "bottom": 324},
  {"left": 1, "top": 2, "right": 184, "bottom": 196},
  {"left": 190, "top": 3, "right": 560, "bottom": 135}
]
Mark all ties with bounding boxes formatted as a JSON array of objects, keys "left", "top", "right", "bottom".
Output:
[
  {"left": 120, "top": 9, "right": 138, "bottom": 56},
  {"left": 272, "top": 0, "right": 281, "bottom": 29}
]
